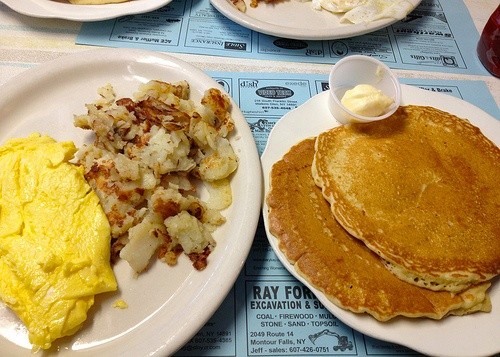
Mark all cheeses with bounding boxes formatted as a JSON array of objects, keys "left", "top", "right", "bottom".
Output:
[
  {"left": 341, "top": 83, "right": 394, "bottom": 116},
  {"left": 0, "top": 133, "right": 129, "bottom": 357}
]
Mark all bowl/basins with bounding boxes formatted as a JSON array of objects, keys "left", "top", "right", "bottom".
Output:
[{"left": 329, "top": 55, "right": 400, "bottom": 122}]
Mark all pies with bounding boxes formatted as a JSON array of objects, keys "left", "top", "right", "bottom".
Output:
[{"left": 268, "top": 105, "right": 500, "bottom": 321}]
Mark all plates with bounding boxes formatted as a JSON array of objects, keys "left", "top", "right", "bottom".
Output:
[
  {"left": 208, "top": 0, "right": 423, "bottom": 41},
  {"left": 259, "top": 83, "right": 499, "bottom": 357},
  {"left": 0, "top": 49, "right": 262, "bottom": 357},
  {"left": 0, "top": 0, "right": 174, "bottom": 21}
]
